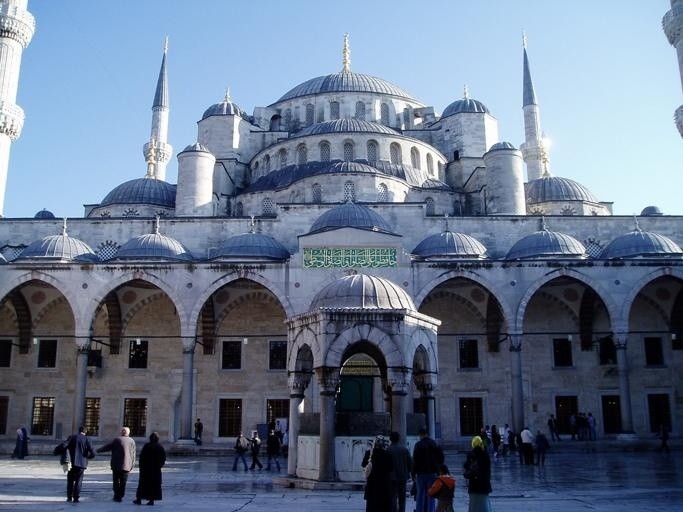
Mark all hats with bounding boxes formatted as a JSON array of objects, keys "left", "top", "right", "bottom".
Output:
[{"left": 472, "top": 436, "right": 482, "bottom": 448}]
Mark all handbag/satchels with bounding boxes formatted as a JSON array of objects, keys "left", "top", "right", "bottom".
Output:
[
  {"left": 362, "top": 462, "right": 372, "bottom": 478},
  {"left": 85, "top": 448, "right": 95, "bottom": 459},
  {"left": 463, "top": 464, "right": 477, "bottom": 479}
]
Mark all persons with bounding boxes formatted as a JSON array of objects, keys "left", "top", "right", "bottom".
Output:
[
  {"left": 264, "top": 428, "right": 281, "bottom": 472},
  {"left": 232, "top": 430, "right": 250, "bottom": 471},
  {"left": 96, "top": 427, "right": 137, "bottom": 502},
  {"left": 361, "top": 423, "right": 548, "bottom": 512},
  {"left": 131, "top": 430, "right": 168, "bottom": 505},
  {"left": 245, "top": 431, "right": 264, "bottom": 471},
  {"left": 54, "top": 425, "right": 95, "bottom": 503},
  {"left": 193, "top": 417, "right": 203, "bottom": 439},
  {"left": 569, "top": 411, "right": 597, "bottom": 441},
  {"left": 548, "top": 413, "right": 561, "bottom": 440},
  {"left": 20, "top": 428, "right": 30, "bottom": 457},
  {"left": 269, "top": 418, "right": 289, "bottom": 458},
  {"left": 11, "top": 428, "right": 25, "bottom": 461}
]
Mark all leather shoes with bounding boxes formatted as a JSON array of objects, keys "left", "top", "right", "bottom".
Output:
[
  {"left": 133, "top": 500, "right": 141, "bottom": 504},
  {"left": 147, "top": 501, "right": 154, "bottom": 505}
]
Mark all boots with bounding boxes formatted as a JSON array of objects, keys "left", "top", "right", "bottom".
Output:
[
  {"left": 74, "top": 496, "right": 79, "bottom": 502},
  {"left": 67, "top": 496, "right": 72, "bottom": 501}
]
